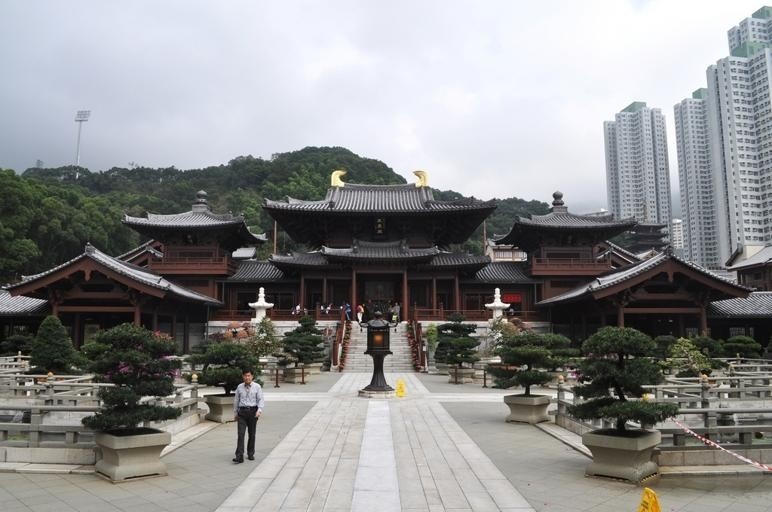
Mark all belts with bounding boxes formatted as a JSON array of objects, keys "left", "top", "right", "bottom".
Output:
[{"left": 240, "top": 407, "right": 257, "bottom": 409}]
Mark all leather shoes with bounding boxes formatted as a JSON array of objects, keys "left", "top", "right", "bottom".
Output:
[
  {"left": 248, "top": 454, "right": 254, "bottom": 460},
  {"left": 232, "top": 456, "right": 243, "bottom": 462}
]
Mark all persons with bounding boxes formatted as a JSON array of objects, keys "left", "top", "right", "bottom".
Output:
[
  {"left": 233, "top": 368, "right": 265, "bottom": 463},
  {"left": 295, "top": 299, "right": 401, "bottom": 324}
]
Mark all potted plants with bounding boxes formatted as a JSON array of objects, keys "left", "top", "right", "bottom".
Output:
[
  {"left": 434, "top": 312, "right": 482, "bottom": 384},
  {"left": 195, "top": 342, "right": 264, "bottom": 424},
  {"left": 78, "top": 323, "right": 184, "bottom": 483},
  {"left": 567, "top": 325, "right": 679, "bottom": 483},
  {"left": 280, "top": 315, "right": 329, "bottom": 375},
  {"left": 484, "top": 331, "right": 581, "bottom": 424}
]
